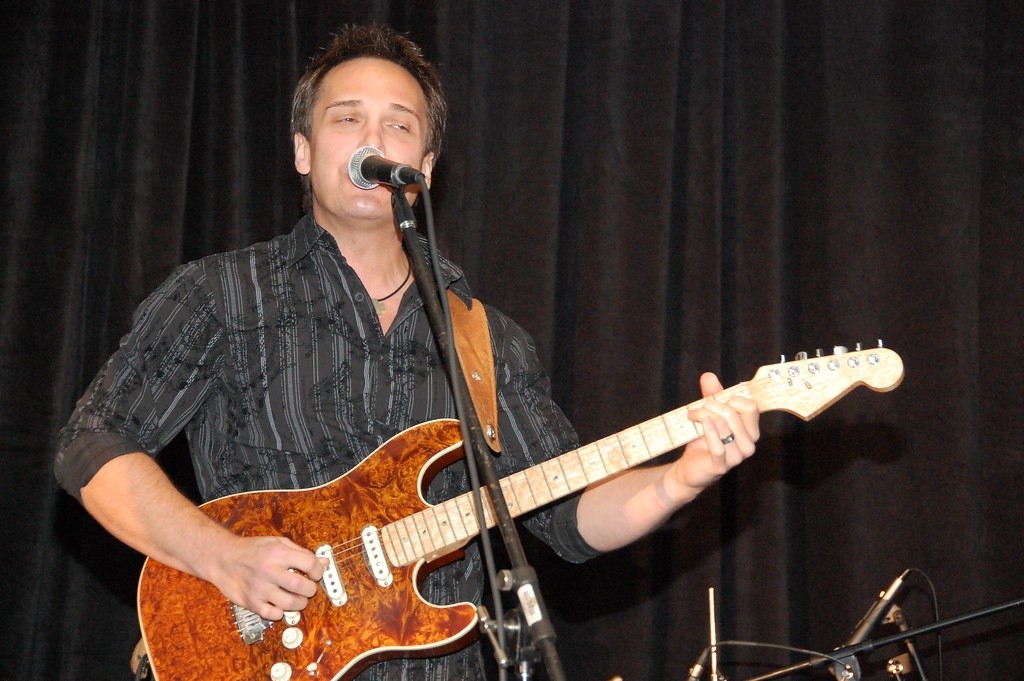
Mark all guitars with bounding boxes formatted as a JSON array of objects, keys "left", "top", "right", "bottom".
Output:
[{"left": 134, "top": 337, "right": 907, "bottom": 681}]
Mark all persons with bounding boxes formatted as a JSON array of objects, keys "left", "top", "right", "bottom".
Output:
[{"left": 51, "top": 23, "right": 761, "bottom": 681}]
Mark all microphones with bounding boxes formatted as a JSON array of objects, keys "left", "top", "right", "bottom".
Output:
[
  {"left": 348, "top": 147, "right": 425, "bottom": 191},
  {"left": 845, "top": 566, "right": 910, "bottom": 648},
  {"left": 687, "top": 647, "right": 710, "bottom": 681}
]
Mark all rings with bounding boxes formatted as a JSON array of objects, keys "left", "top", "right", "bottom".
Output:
[{"left": 722, "top": 433, "right": 735, "bottom": 444}]
